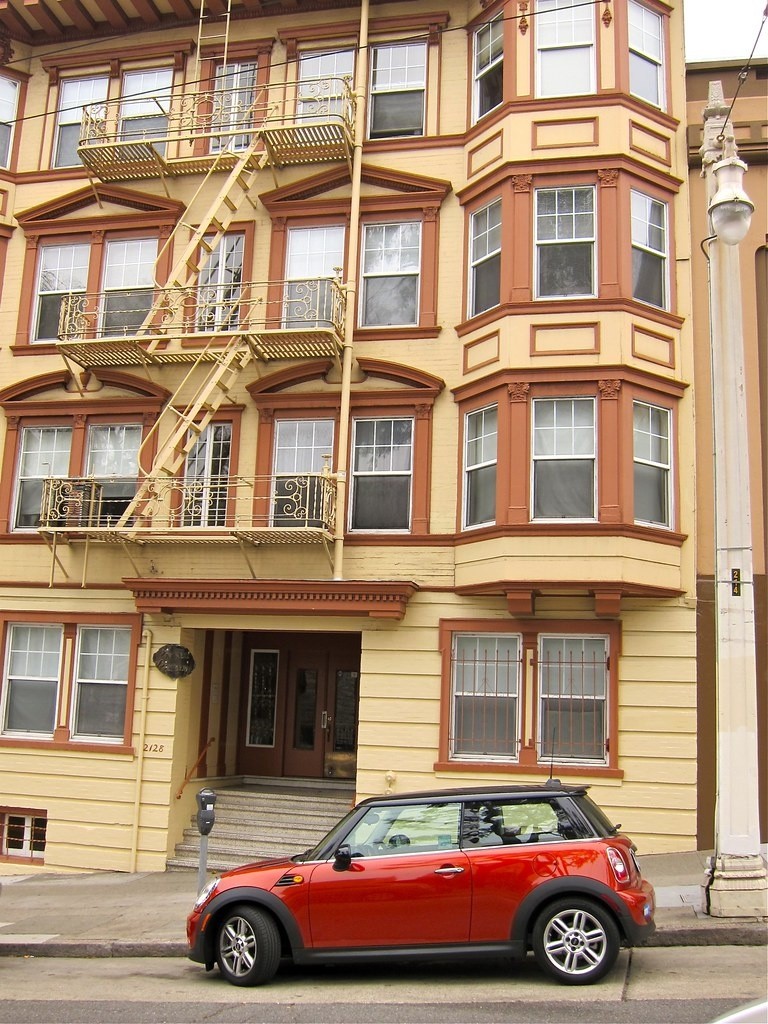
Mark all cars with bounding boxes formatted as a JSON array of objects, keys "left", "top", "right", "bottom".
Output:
[{"left": 187, "top": 779, "right": 656, "bottom": 987}]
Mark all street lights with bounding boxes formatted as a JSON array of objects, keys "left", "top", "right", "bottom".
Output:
[{"left": 706, "top": 156, "right": 767, "bottom": 919}]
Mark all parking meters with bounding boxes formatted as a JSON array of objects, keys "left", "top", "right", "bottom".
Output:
[{"left": 194, "top": 788, "right": 217, "bottom": 896}]
